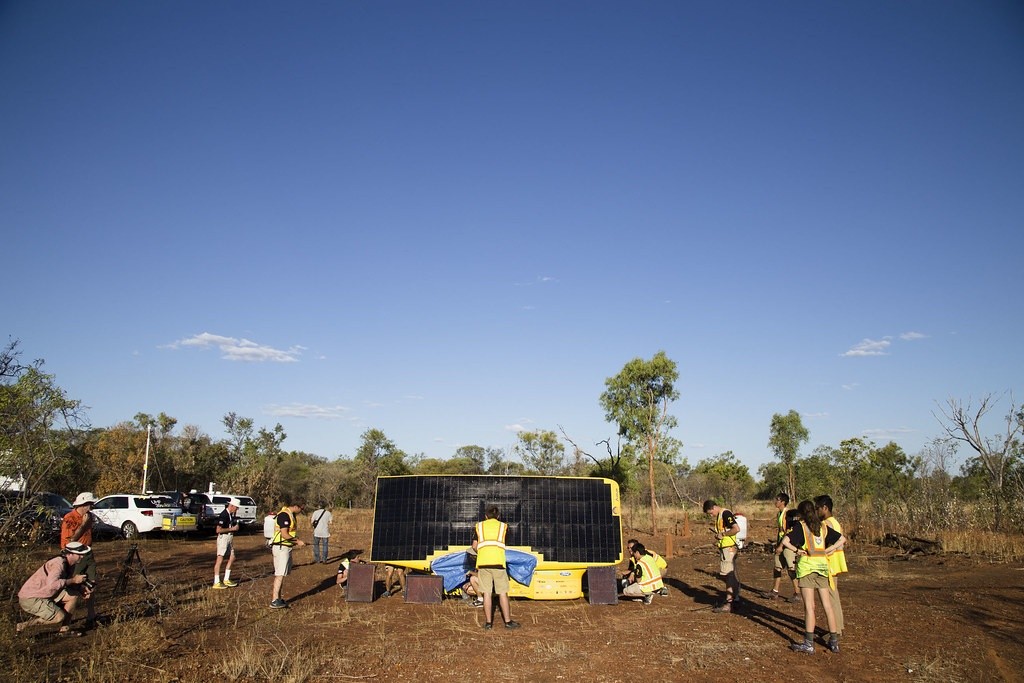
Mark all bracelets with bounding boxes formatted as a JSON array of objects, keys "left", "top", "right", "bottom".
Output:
[
  {"left": 722, "top": 532, "right": 725, "bottom": 536},
  {"left": 794, "top": 547, "right": 798, "bottom": 554}
]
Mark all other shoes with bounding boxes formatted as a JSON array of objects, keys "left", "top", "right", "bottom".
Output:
[
  {"left": 645, "top": 595, "right": 654, "bottom": 605},
  {"left": 661, "top": 588, "right": 668, "bottom": 596},
  {"left": 381, "top": 592, "right": 391, "bottom": 598}
]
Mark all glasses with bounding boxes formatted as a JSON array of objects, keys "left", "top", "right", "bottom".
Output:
[{"left": 298, "top": 506, "right": 304, "bottom": 510}]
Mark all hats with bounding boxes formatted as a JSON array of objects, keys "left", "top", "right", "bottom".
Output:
[
  {"left": 66, "top": 542, "right": 92, "bottom": 554},
  {"left": 73, "top": 492, "right": 99, "bottom": 505},
  {"left": 227, "top": 498, "right": 242, "bottom": 508}
]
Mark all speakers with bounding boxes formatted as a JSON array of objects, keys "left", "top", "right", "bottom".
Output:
[
  {"left": 345, "top": 563, "right": 376, "bottom": 602},
  {"left": 405, "top": 575, "right": 445, "bottom": 604},
  {"left": 588, "top": 565, "right": 618, "bottom": 604}
]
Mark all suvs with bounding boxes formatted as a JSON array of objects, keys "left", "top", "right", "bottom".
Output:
[
  {"left": 88, "top": 490, "right": 182, "bottom": 541},
  {"left": 0, "top": 488, "right": 75, "bottom": 544},
  {"left": 147, "top": 482, "right": 258, "bottom": 539}
]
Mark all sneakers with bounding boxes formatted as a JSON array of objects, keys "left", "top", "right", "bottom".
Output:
[
  {"left": 713, "top": 605, "right": 731, "bottom": 613},
  {"left": 213, "top": 582, "right": 228, "bottom": 589},
  {"left": 787, "top": 595, "right": 803, "bottom": 602},
  {"left": 505, "top": 621, "right": 521, "bottom": 629},
  {"left": 828, "top": 639, "right": 839, "bottom": 653},
  {"left": 269, "top": 599, "right": 288, "bottom": 608},
  {"left": 223, "top": 580, "right": 237, "bottom": 587},
  {"left": 469, "top": 601, "right": 484, "bottom": 607},
  {"left": 483, "top": 624, "right": 491, "bottom": 629},
  {"left": 791, "top": 642, "right": 814, "bottom": 653},
  {"left": 761, "top": 591, "right": 778, "bottom": 599}
]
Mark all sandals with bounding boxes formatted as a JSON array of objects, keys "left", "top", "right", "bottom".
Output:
[{"left": 60, "top": 629, "right": 80, "bottom": 638}]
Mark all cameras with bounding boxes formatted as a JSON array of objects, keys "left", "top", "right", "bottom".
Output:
[{"left": 82, "top": 573, "right": 97, "bottom": 591}]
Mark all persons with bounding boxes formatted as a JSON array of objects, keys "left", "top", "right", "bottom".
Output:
[
  {"left": 211, "top": 498, "right": 241, "bottom": 589},
  {"left": 761, "top": 492, "right": 804, "bottom": 601},
  {"left": 310, "top": 501, "right": 334, "bottom": 564},
  {"left": 472, "top": 505, "right": 522, "bottom": 629},
  {"left": 16, "top": 542, "right": 95, "bottom": 637},
  {"left": 269, "top": 498, "right": 307, "bottom": 609},
  {"left": 782, "top": 500, "right": 847, "bottom": 654},
  {"left": 813, "top": 494, "right": 848, "bottom": 637},
  {"left": 463, "top": 547, "right": 484, "bottom": 608},
  {"left": 380, "top": 563, "right": 406, "bottom": 598},
  {"left": 336, "top": 549, "right": 366, "bottom": 598},
  {"left": 60, "top": 492, "right": 98, "bottom": 629},
  {"left": 622, "top": 539, "right": 668, "bottom": 605},
  {"left": 702, "top": 499, "right": 740, "bottom": 612}
]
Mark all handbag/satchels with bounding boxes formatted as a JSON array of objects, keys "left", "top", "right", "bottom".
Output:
[{"left": 312, "top": 520, "right": 319, "bottom": 528}]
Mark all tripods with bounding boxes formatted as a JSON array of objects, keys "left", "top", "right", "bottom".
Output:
[{"left": 112, "top": 544, "right": 150, "bottom": 595}]
